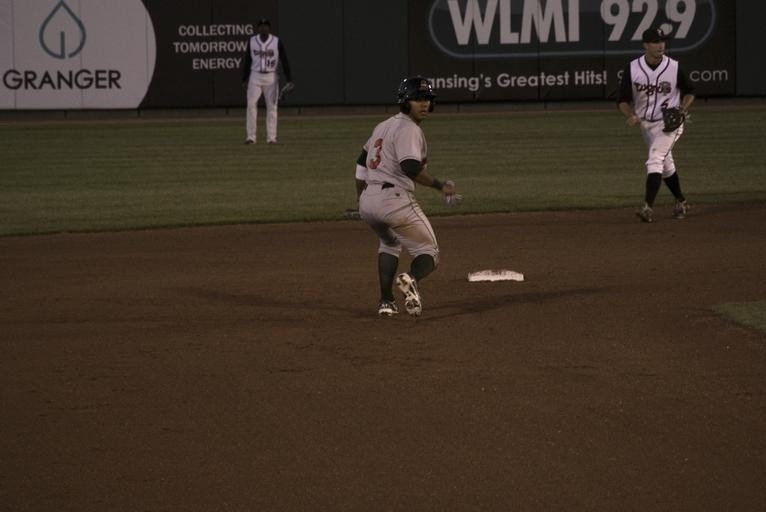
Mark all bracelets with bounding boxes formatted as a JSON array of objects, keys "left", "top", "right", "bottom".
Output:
[{"left": 431, "top": 178, "right": 445, "bottom": 190}]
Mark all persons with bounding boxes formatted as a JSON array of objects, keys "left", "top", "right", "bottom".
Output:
[
  {"left": 240, "top": 19, "right": 293, "bottom": 144},
  {"left": 617, "top": 27, "right": 699, "bottom": 223},
  {"left": 355, "top": 77, "right": 456, "bottom": 316}
]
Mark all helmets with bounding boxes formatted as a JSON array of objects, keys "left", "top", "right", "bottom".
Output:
[
  {"left": 398, "top": 78, "right": 437, "bottom": 104},
  {"left": 257, "top": 18, "right": 270, "bottom": 26}
]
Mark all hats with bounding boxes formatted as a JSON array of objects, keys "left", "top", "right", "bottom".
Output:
[{"left": 641, "top": 27, "right": 670, "bottom": 43}]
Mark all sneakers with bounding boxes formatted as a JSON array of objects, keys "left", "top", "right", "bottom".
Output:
[
  {"left": 396, "top": 273, "right": 423, "bottom": 316},
  {"left": 378, "top": 299, "right": 399, "bottom": 317},
  {"left": 673, "top": 198, "right": 690, "bottom": 219},
  {"left": 246, "top": 136, "right": 254, "bottom": 144},
  {"left": 635, "top": 203, "right": 653, "bottom": 222},
  {"left": 267, "top": 138, "right": 277, "bottom": 143}
]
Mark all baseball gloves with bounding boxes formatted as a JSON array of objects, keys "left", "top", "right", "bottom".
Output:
[{"left": 661, "top": 108, "right": 685, "bottom": 132}]
